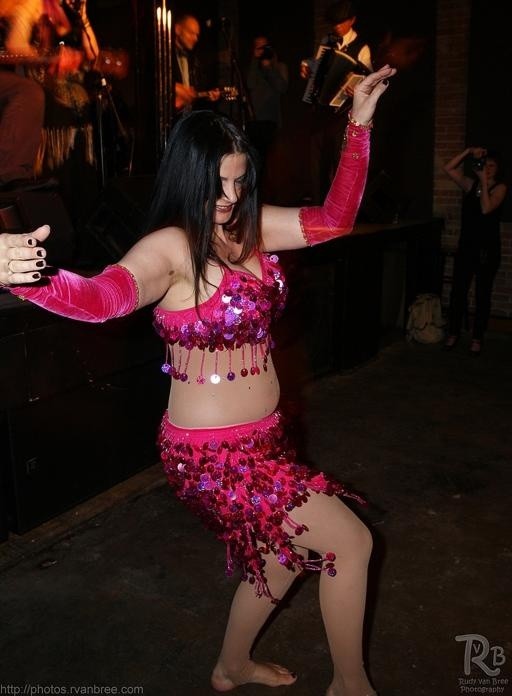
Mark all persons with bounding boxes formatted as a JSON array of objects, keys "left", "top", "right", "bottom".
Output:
[
  {"left": 1, "top": 1, "right": 378, "bottom": 173},
  {"left": 442, "top": 145, "right": 507, "bottom": 360},
  {"left": 1, "top": 63, "right": 399, "bottom": 696}
]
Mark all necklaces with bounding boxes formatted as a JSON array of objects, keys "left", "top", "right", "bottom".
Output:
[{"left": 476, "top": 183, "right": 483, "bottom": 196}]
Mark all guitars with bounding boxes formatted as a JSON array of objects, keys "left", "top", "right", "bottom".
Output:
[{"left": 176, "top": 83, "right": 239, "bottom": 106}]
